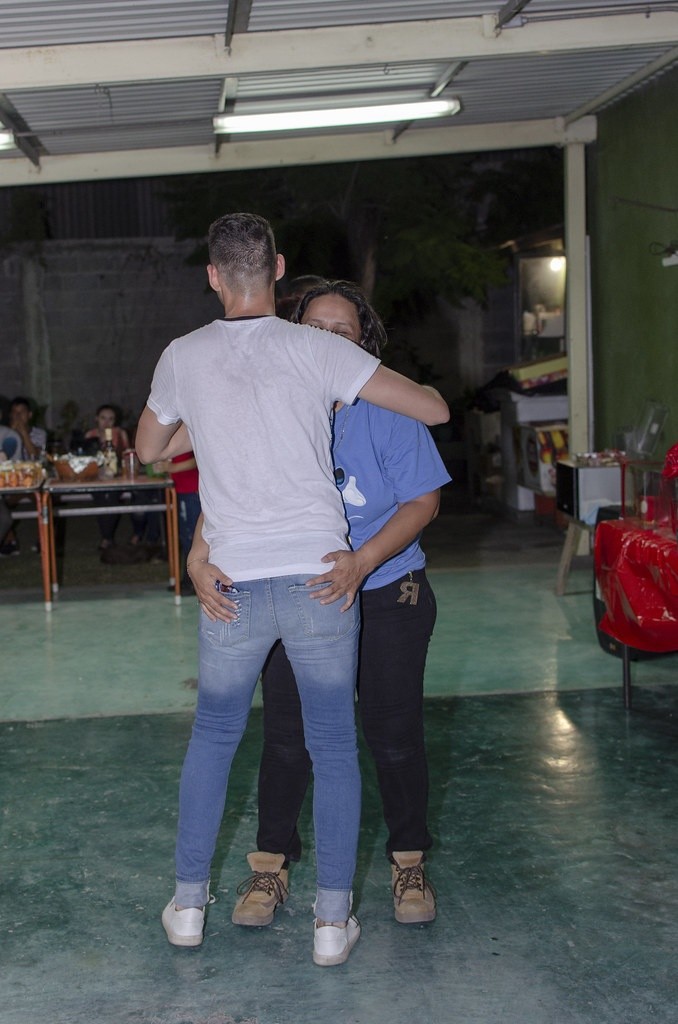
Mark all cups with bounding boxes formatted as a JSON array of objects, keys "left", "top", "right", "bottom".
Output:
[{"left": 125, "top": 450, "right": 139, "bottom": 479}]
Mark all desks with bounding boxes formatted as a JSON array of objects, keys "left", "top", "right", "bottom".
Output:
[
  {"left": 0, "top": 472, "right": 183, "bottom": 611},
  {"left": 592, "top": 518, "right": 678, "bottom": 708}
]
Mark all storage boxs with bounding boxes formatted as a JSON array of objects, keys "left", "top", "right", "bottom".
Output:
[
  {"left": 500, "top": 394, "right": 571, "bottom": 424},
  {"left": 521, "top": 311, "right": 566, "bottom": 338}
]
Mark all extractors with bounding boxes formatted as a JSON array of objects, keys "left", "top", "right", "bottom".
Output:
[{"left": 518, "top": 229, "right": 566, "bottom": 259}]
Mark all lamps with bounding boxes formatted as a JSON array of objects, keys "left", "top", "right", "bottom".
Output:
[{"left": 212, "top": 96, "right": 462, "bottom": 134}]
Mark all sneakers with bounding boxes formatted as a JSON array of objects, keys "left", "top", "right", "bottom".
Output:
[
  {"left": 391, "top": 851, "right": 439, "bottom": 922},
  {"left": 232, "top": 851, "right": 290, "bottom": 925},
  {"left": 313, "top": 914, "right": 361, "bottom": 965},
  {"left": 162, "top": 893, "right": 216, "bottom": 946}
]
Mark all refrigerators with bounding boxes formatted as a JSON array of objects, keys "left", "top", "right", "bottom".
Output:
[{"left": 479, "top": 257, "right": 522, "bottom": 387}]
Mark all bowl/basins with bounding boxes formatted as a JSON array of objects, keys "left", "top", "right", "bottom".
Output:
[
  {"left": 54, "top": 458, "right": 97, "bottom": 481},
  {"left": 576, "top": 453, "right": 623, "bottom": 466}
]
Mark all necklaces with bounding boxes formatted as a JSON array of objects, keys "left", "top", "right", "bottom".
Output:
[{"left": 330, "top": 404, "right": 348, "bottom": 452}]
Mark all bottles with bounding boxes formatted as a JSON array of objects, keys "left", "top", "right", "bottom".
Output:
[
  {"left": 0, "top": 461, "right": 44, "bottom": 487},
  {"left": 102, "top": 429, "right": 117, "bottom": 477}
]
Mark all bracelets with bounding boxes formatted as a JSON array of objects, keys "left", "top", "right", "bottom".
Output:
[{"left": 187, "top": 558, "right": 206, "bottom": 578}]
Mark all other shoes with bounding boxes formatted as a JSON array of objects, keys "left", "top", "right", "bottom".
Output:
[{"left": 1, "top": 540, "right": 19, "bottom": 557}]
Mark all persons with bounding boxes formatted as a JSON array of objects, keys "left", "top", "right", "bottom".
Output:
[
  {"left": 131, "top": 490, "right": 164, "bottom": 546},
  {"left": 187, "top": 280, "right": 454, "bottom": 928},
  {"left": 159, "top": 450, "right": 202, "bottom": 596},
  {"left": 136, "top": 213, "right": 450, "bottom": 966},
  {"left": 0, "top": 397, "right": 48, "bottom": 556},
  {"left": 87, "top": 404, "right": 130, "bottom": 551}
]
